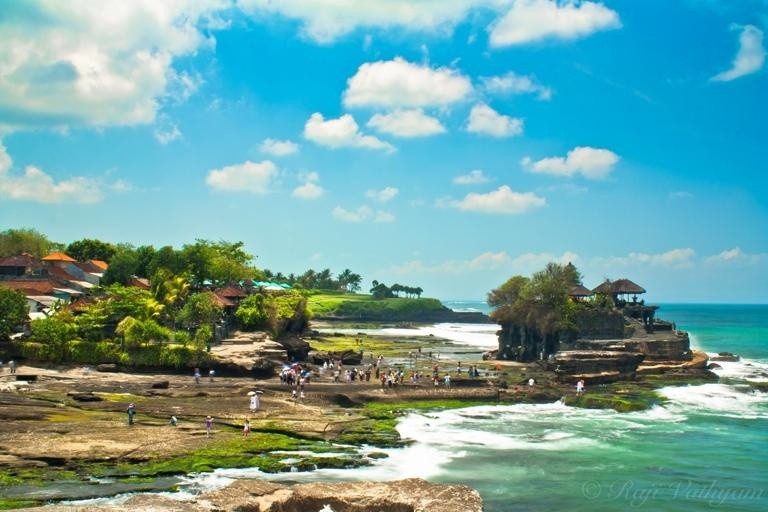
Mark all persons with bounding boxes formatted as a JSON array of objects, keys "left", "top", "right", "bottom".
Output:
[
  {"left": 168, "top": 415, "right": 177, "bottom": 426},
  {"left": 126, "top": 403, "right": 136, "bottom": 425},
  {"left": 241, "top": 417, "right": 251, "bottom": 440},
  {"left": 7, "top": 358, "right": 18, "bottom": 374},
  {"left": 575, "top": 379, "right": 586, "bottom": 397},
  {"left": 248, "top": 394, "right": 256, "bottom": 413},
  {"left": 277, "top": 336, "right": 536, "bottom": 401},
  {"left": 205, "top": 415, "right": 213, "bottom": 439},
  {"left": 208, "top": 367, "right": 217, "bottom": 382},
  {"left": 0, "top": 360, "right": 5, "bottom": 373},
  {"left": 191, "top": 367, "right": 202, "bottom": 385}
]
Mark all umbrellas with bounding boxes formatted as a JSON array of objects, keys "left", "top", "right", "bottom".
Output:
[
  {"left": 254, "top": 390, "right": 264, "bottom": 395},
  {"left": 247, "top": 391, "right": 256, "bottom": 396},
  {"left": 257, "top": 394, "right": 261, "bottom": 409}
]
